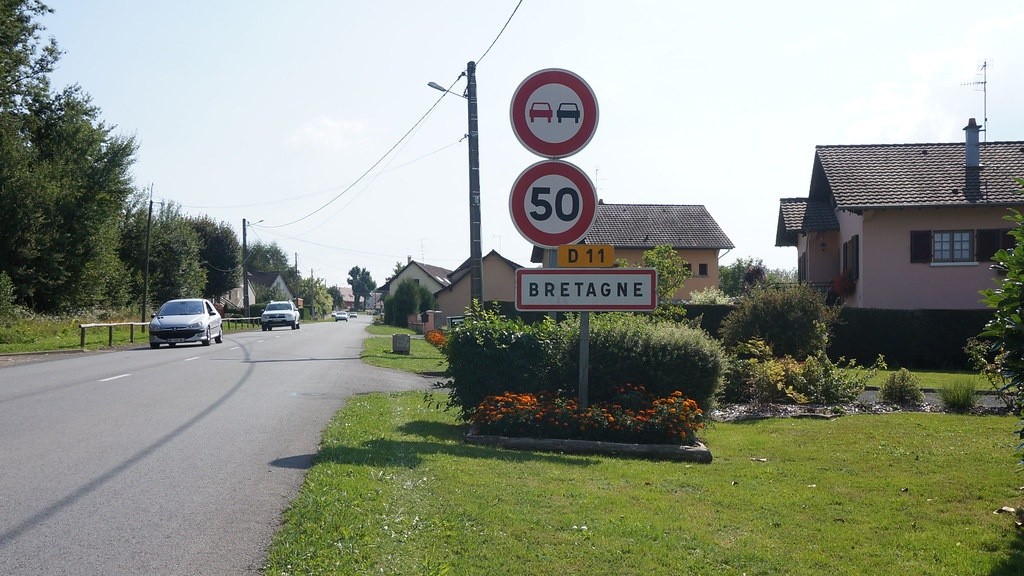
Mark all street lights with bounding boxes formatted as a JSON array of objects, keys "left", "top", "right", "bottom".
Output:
[
  {"left": 428, "top": 81, "right": 484, "bottom": 312},
  {"left": 243, "top": 219, "right": 264, "bottom": 317}
]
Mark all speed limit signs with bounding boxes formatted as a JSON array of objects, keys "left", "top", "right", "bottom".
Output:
[{"left": 508, "top": 159, "right": 598, "bottom": 249}]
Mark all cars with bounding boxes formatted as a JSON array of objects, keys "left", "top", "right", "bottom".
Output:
[
  {"left": 331, "top": 311, "right": 337, "bottom": 317},
  {"left": 335, "top": 312, "right": 348, "bottom": 322},
  {"left": 349, "top": 312, "right": 357, "bottom": 318},
  {"left": 149, "top": 299, "right": 223, "bottom": 350}
]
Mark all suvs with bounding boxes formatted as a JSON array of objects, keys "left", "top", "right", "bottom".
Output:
[{"left": 261, "top": 300, "right": 300, "bottom": 331}]
